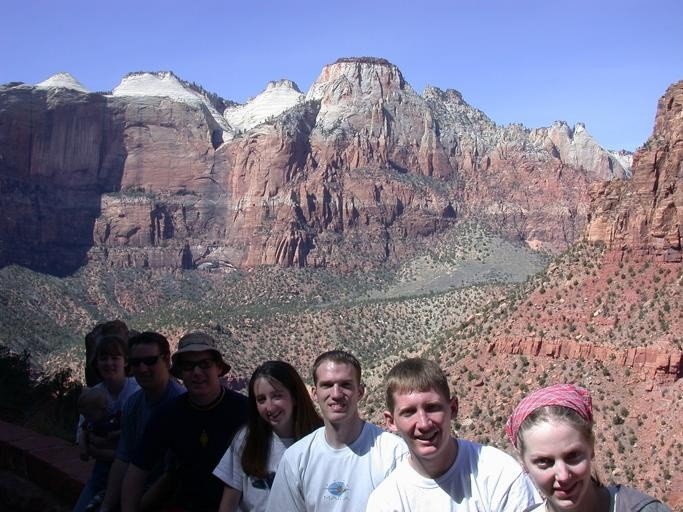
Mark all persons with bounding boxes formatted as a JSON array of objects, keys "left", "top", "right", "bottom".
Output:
[
  {"left": 266, "top": 348, "right": 410, "bottom": 512},
  {"left": 62, "top": 333, "right": 143, "bottom": 512},
  {"left": 366, "top": 357, "right": 544, "bottom": 512},
  {"left": 97, "top": 330, "right": 188, "bottom": 512},
  {"left": 75, "top": 387, "right": 125, "bottom": 510},
  {"left": 120, "top": 330, "right": 251, "bottom": 512},
  {"left": 505, "top": 383, "right": 675, "bottom": 512},
  {"left": 212, "top": 360, "right": 325, "bottom": 512}
]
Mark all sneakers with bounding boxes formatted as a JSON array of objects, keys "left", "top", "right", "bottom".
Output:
[{"left": 85, "top": 500, "right": 101, "bottom": 512}]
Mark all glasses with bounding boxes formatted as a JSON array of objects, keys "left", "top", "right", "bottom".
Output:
[
  {"left": 131, "top": 354, "right": 160, "bottom": 368},
  {"left": 180, "top": 358, "right": 217, "bottom": 370}
]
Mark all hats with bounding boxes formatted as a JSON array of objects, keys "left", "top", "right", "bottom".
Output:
[{"left": 169, "top": 333, "right": 231, "bottom": 380}]
